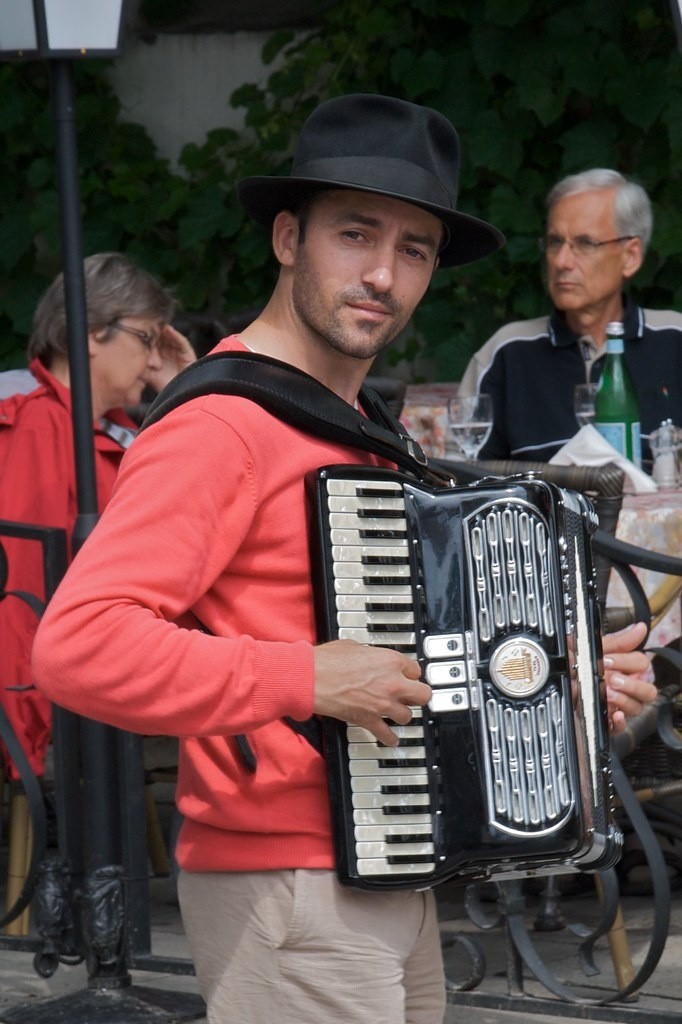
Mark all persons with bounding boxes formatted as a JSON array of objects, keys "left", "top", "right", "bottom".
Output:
[
  {"left": 28, "top": 92, "right": 659, "bottom": 1024},
  {"left": 445, "top": 168, "right": 682, "bottom": 904},
  {"left": 0, "top": 252, "right": 198, "bottom": 783}
]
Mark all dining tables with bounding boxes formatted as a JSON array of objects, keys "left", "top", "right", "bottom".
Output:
[
  {"left": 353, "top": 379, "right": 460, "bottom": 460},
  {"left": 571, "top": 481, "right": 682, "bottom": 659}
]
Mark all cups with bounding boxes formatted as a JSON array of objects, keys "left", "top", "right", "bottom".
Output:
[
  {"left": 447, "top": 392, "right": 494, "bottom": 460},
  {"left": 571, "top": 382, "right": 600, "bottom": 430}
]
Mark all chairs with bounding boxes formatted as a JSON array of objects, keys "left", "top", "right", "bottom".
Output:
[{"left": 437, "top": 460, "right": 682, "bottom": 1003}]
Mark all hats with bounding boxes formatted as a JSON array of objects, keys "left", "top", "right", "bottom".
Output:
[{"left": 235, "top": 92, "right": 508, "bottom": 268}]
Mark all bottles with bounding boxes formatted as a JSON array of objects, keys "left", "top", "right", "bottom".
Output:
[{"left": 594, "top": 321, "right": 641, "bottom": 471}]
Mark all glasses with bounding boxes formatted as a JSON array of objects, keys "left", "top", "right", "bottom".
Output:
[
  {"left": 538, "top": 235, "right": 635, "bottom": 256},
  {"left": 107, "top": 320, "right": 164, "bottom": 349}
]
